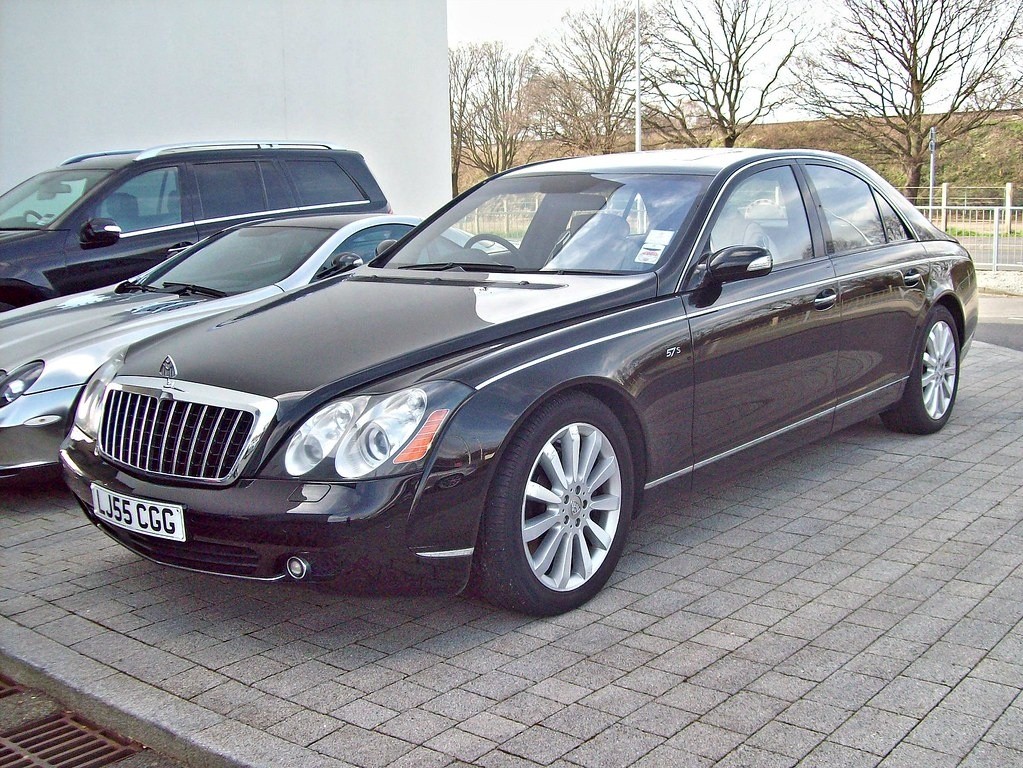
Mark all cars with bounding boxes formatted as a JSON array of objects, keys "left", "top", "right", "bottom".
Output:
[{"left": 59, "top": 148, "right": 980, "bottom": 615}]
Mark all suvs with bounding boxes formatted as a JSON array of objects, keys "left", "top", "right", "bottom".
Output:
[{"left": 2, "top": 143, "right": 391, "bottom": 312}]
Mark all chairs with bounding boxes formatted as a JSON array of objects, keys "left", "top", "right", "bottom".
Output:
[
  {"left": 547, "top": 213, "right": 638, "bottom": 270},
  {"left": 106, "top": 192, "right": 143, "bottom": 222},
  {"left": 160, "top": 191, "right": 181, "bottom": 223},
  {"left": 708, "top": 202, "right": 785, "bottom": 265}
]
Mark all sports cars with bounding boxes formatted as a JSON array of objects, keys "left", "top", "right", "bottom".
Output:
[{"left": 0, "top": 212, "right": 498, "bottom": 475}]
